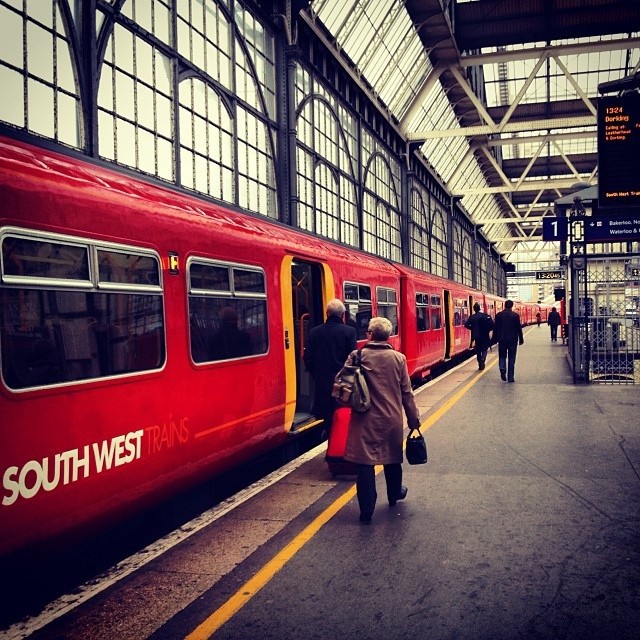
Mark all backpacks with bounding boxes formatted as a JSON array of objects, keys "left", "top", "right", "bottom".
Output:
[{"left": 333, "top": 350, "right": 370, "bottom": 411}]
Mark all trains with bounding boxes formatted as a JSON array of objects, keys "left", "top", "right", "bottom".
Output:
[{"left": 1, "top": 136, "right": 561, "bottom": 556}]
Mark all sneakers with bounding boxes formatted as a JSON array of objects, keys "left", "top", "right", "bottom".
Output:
[
  {"left": 554, "top": 338, "right": 557, "bottom": 342},
  {"left": 551, "top": 338, "right": 553, "bottom": 341},
  {"left": 482, "top": 357, "right": 485, "bottom": 369},
  {"left": 478, "top": 363, "right": 483, "bottom": 370},
  {"left": 500, "top": 369, "right": 507, "bottom": 381},
  {"left": 508, "top": 378, "right": 515, "bottom": 383}
]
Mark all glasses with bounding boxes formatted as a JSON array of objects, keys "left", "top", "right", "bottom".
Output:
[{"left": 365, "top": 331, "right": 372, "bottom": 335}]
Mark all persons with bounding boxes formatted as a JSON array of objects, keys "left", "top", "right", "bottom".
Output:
[
  {"left": 83, "top": 307, "right": 132, "bottom": 375},
  {"left": 209, "top": 308, "right": 253, "bottom": 358},
  {"left": 536, "top": 311, "right": 541, "bottom": 327},
  {"left": 495, "top": 300, "right": 524, "bottom": 383},
  {"left": 548, "top": 307, "right": 561, "bottom": 341},
  {"left": 341, "top": 316, "right": 420, "bottom": 523},
  {"left": 465, "top": 302, "right": 495, "bottom": 369},
  {"left": 305, "top": 299, "right": 357, "bottom": 442}
]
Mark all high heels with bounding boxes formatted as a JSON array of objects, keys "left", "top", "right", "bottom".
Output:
[
  {"left": 359, "top": 512, "right": 370, "bottom": 524},
  {"left": 387, "top": 487, "right": 407, "bottom": 508}
]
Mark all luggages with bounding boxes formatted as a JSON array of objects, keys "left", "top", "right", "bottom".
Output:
[{"left": 324, "top": 407, "right": 360, "bottom": 479}]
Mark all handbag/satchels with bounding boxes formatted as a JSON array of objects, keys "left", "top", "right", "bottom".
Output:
[
  {"left": 477, "top": 313, "right": 494, "bottom": 333},
  {"left": 407, "top": 428, "right": 428, "bottom": 464}
]
